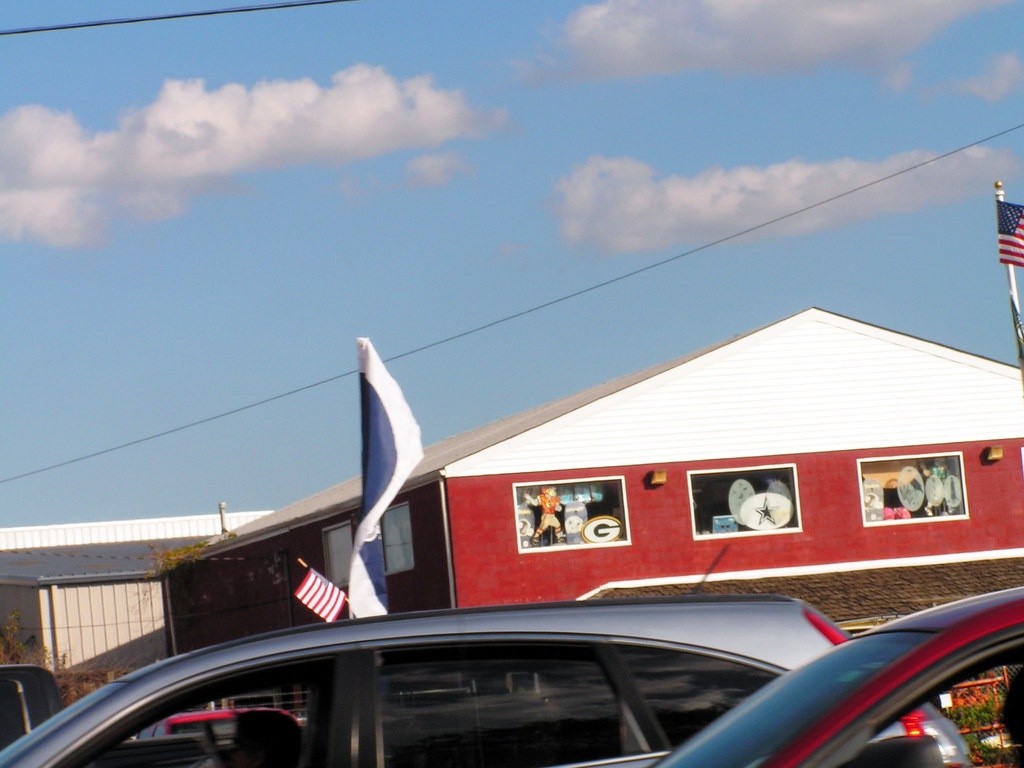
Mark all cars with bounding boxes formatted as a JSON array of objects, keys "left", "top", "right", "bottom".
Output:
[
  {"left": 1, "top": 587, "right": 974, "bottom": 768},
  {"left": 646, "top": 585, "right": 1024, "bottom": 768}
]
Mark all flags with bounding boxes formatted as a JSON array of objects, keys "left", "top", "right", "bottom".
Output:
[
  {"left": 997, "top": 200, "right": 1024, "bottom": 268},
  {"left": 345, "top": 343, "right": 424, "bottom": 619},
  {"left": 294, "top": 568, "right": 346, "bottom": 622}
]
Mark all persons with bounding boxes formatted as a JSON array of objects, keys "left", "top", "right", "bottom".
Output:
[{"left": 231, "top": 710, "right": 302, "bottom": 768}]
